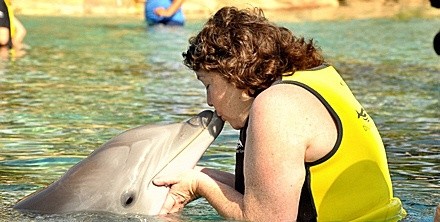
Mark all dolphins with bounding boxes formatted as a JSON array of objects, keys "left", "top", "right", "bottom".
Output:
[{"left": 12, "top": 110, "right": 222, "bottom": 216}]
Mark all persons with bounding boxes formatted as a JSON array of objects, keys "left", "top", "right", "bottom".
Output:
[
  {"left": 0, "top": 0, "right": 27, "bottom": 48},
  {"left": 145, "top": 0, "right": 185, "bottom": 28},
  {"left": 152, "top": 7, "right": 406, "bottom": 222}
]
